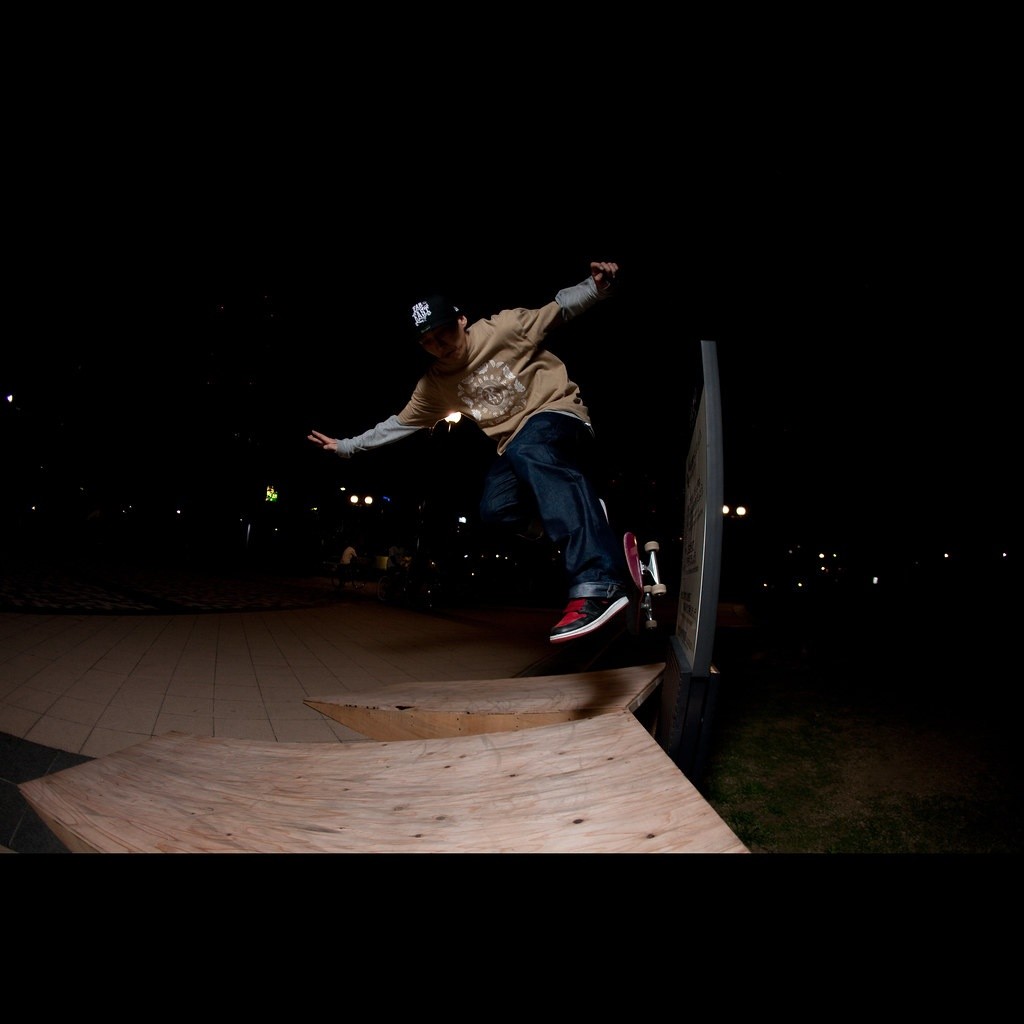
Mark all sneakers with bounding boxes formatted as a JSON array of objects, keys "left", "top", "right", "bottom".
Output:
[
  {"left": 594, "top": 496, "right": 611, "bottom": 526},
  {"left": 548, "top": 587, "right": 631, "bottom": 645}
]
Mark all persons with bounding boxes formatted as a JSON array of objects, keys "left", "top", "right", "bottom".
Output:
[
  {"left": 385, "top": 543, "right": 402, "bottom": 576},
  {"left": 307, "top": 260, "right": 630, "bottom": 644},
  {"left": 336, "top": 543, "right": 357, "bottom": 590}
]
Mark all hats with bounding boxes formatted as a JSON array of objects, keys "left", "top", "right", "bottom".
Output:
[{"left": 404, "top": 294, "right": 464, "bottom": 346}]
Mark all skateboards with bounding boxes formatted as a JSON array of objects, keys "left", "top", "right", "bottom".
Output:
[{"left": 621, "top": 530, "right": 669, "bottom": 632}]
